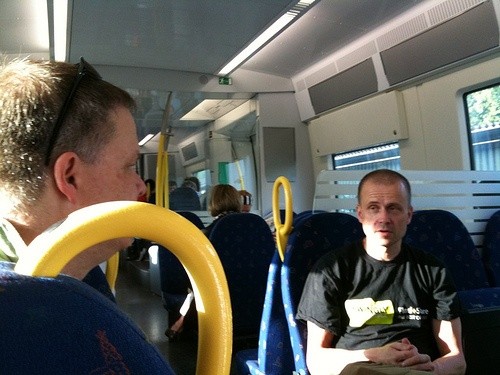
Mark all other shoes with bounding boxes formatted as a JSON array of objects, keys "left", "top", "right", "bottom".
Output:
[{"left": 165, "top": 325, "right": 177, "bottom": 339}]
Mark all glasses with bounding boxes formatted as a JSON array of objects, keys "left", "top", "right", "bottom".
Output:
[{"left": 43, "top": 56, "right": 102, "bottom": 165}]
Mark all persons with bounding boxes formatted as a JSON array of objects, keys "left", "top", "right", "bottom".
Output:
[
  {"left": 298, "top": 169, "right": 466, "bottom": 375},
  {"left": 0, "top": 54, "right": 146, "bottom": 304},
  {"left": 106, "top": 173, "right": 385, "bottom": 334}
]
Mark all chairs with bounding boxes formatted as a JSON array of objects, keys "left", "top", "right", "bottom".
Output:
[{"left": 0, "top": 186, "right": 500, "bottom": 375}]
